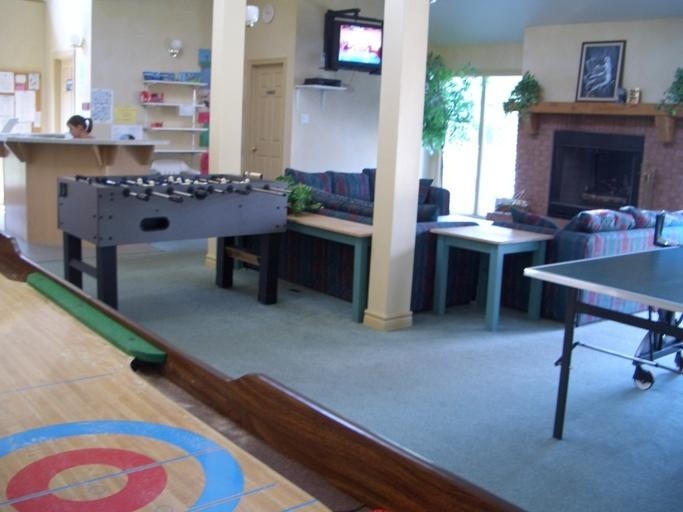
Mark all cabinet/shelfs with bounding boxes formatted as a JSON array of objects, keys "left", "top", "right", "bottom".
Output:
[{"left": 143, "top": 79, "right": 209, "bottom": 154}]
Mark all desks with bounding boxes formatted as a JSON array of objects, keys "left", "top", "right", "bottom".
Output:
[
  {"left": 1, "top": 231, "right": 523, "bottom": 512},
  {"left": 428, "top": 224, "right": 554, "bottom": 330},
  {"left": 234, "top": 212, "right": 373, "bottom": 322},
  {"left": 523, "top": 245, "right": 682, "bottom": 440}
]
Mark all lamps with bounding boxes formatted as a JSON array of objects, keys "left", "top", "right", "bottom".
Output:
[
  {"left": 246, "top": 6, "right": 258, "bottom": 28},
  {"left": 168, "top": 39, "right": 182, "bottom": 58}
]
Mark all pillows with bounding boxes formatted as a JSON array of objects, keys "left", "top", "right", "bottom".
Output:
[
  {"left": 498, "top": 205, "right": 683, "bottom": 233},
  {"left": 286, "top": 167, "right": 439, "bottom": 222}
]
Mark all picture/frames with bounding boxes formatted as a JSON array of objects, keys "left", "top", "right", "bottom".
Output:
[{"left": 575, "top": 40, "right": 626, "bottom": 103}]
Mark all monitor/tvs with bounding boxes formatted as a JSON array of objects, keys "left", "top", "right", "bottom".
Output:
[{"left": 330, "top": 22, "right": 383, "bottom": 72}]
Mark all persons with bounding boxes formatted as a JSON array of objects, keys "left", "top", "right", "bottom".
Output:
[{"left": 65, "top": 115, "right": 96, "bottom": 138}]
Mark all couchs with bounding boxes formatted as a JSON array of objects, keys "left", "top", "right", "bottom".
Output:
[
  {"left": 243, "top": 187, "right": 479, "bottom": 313},
  {"left": 491, "top": 210, "right": 683, "bottom": 325}
]
